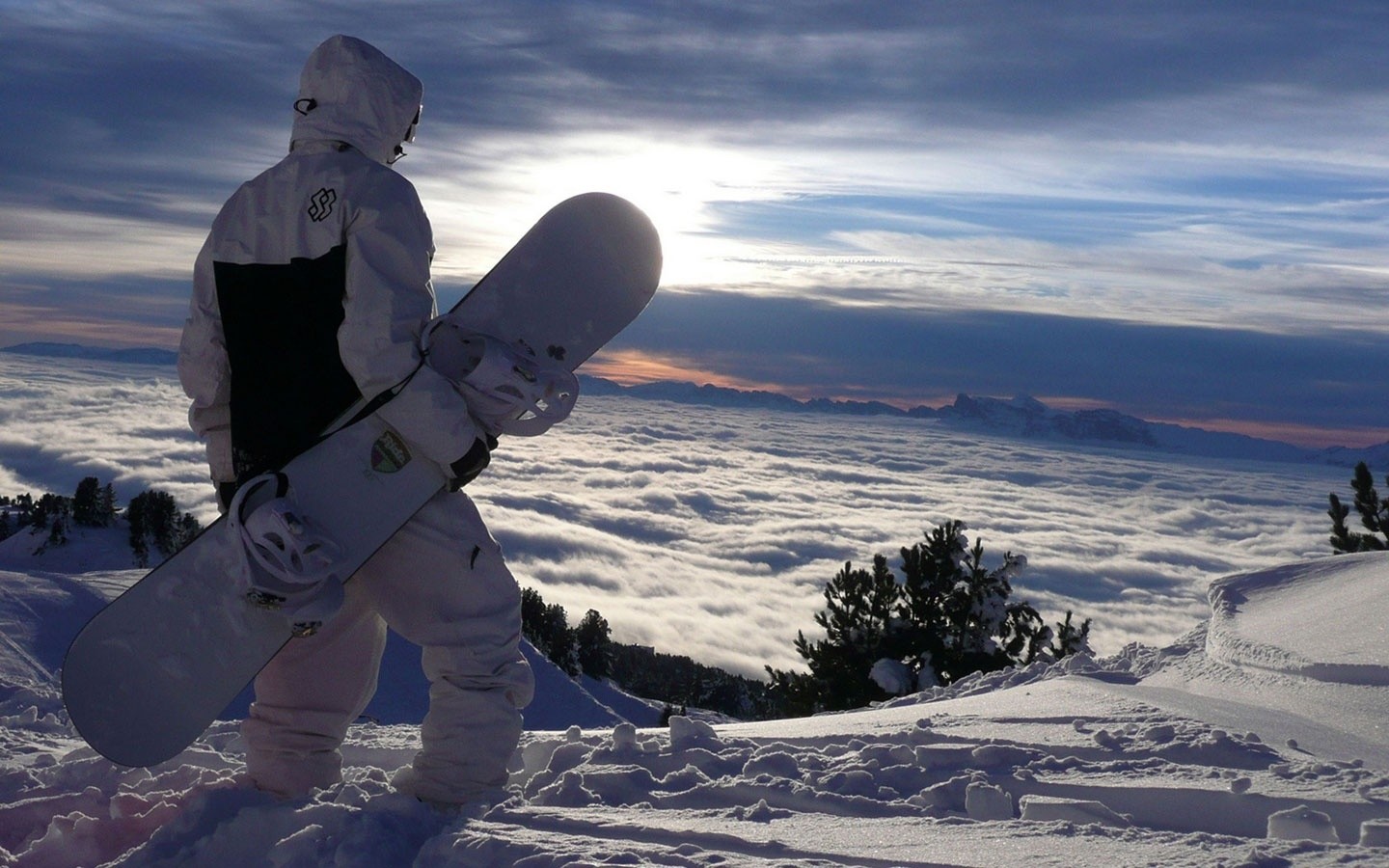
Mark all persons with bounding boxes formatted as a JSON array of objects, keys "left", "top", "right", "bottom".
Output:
[{"left": 178, "top": 34, "right": 534, "bottom": 801}]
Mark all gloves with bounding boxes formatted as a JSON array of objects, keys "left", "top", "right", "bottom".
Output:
[
  {"left": 435, "top": 424, "right": 500, "bottom": 494},
  {"left": 213, "top": 479, "right": 237, "bottom": 516}
]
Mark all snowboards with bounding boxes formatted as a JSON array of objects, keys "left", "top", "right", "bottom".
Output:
[{"left": 60, "top": 193, "right": 664, "bottom": 766}]
